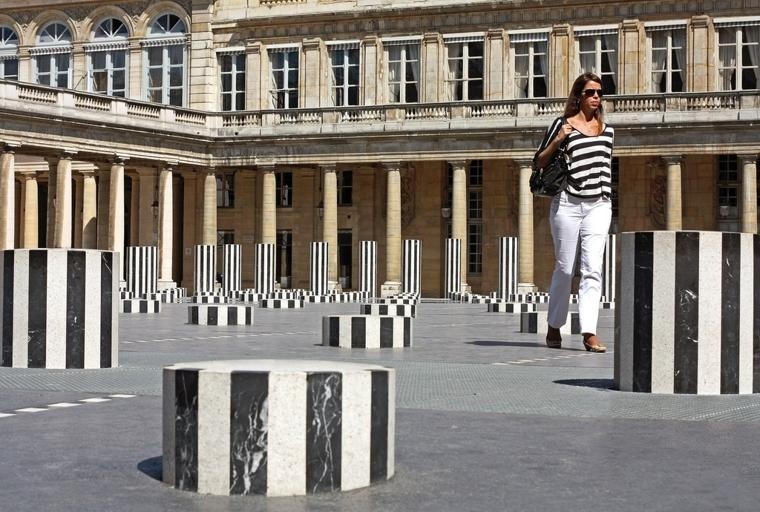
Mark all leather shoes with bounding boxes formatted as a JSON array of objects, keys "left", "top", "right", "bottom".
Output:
[
  {"left": 547, "top": 335, "right": 561, "bottom": 348},
  {"left": 583, "top": 339, "right": 607, "bottom": 352}
]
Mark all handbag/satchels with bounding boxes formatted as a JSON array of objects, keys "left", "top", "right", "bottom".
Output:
[{"left": 529, "top": 117, "right": 570, "bottom": 198}]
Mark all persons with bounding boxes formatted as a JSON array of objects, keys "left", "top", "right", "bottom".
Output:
[{"left": 531, "top": 72, "right": 617, "bottom": 354}]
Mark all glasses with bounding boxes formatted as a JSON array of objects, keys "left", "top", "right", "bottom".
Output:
[{"left": 581, "top": 89, "right": 603, "bottom": 97}]
